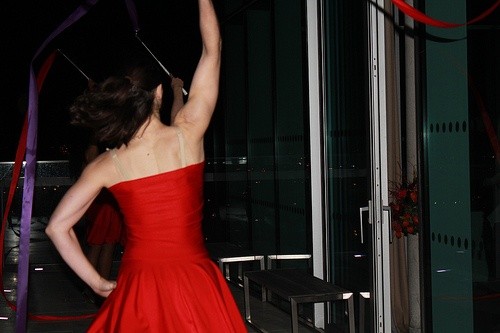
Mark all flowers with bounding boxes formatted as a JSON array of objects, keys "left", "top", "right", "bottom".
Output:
[{"left": 391, "top": 180, "right": 425, "bottom": 241}]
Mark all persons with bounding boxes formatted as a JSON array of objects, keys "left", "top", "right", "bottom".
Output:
[{"left": 44, "top": 0, "right": 253, "bottom": 333}]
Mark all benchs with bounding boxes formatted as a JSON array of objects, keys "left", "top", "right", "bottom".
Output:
[
  {"left": 206, "top": 241, "right": 266, "bottom": 284},
  {"left": 242, "top": 266, "right": 357, "bottom": 333}
]
